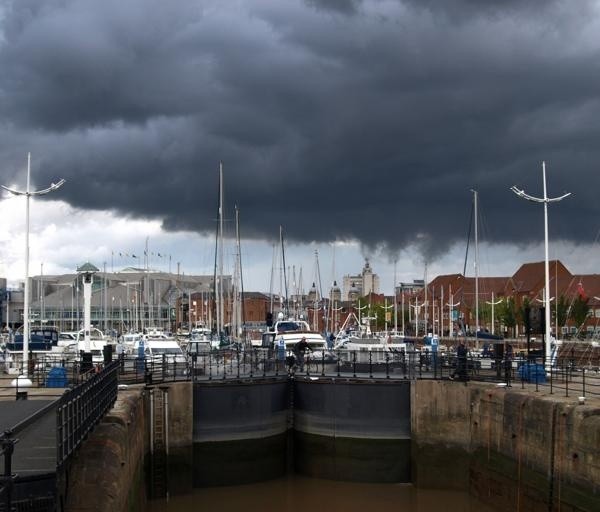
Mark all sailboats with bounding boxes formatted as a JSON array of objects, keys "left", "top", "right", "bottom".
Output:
[
  {"left": 0, "top": 151, "right": 416, "bottom": 377},
  {"left": 420, "top": 187, "right": 528, "bottom": 370}
]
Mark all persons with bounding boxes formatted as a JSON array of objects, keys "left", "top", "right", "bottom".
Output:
[
  {"left": 12, "top": 371, "right": 33, "bottom": 401},
  {"left": 294, "top": 336, "right": 314, "bottom": 373},
  {"left": 448, "top": 339, "right": 471, "bottom": 381}
]
